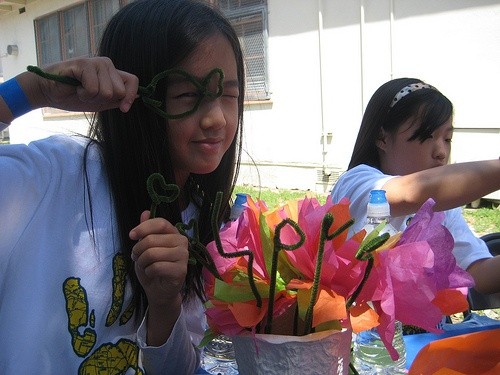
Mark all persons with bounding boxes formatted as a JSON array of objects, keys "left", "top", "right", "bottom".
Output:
[
  {"left": 0, "top": 0, "right": 246, "bottom": 375},
  {"left": 331, "top": 77, "right": 500, "bottom": 370}
]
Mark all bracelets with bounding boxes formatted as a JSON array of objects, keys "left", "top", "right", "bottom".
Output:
[{"left": 0, "top": 76, "right": 33, "bottom": 120}]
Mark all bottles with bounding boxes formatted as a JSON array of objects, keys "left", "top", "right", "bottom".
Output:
[
  {"left": 354, "top": 190, "right": 406, "bottom": 375},
  {"left": 199, "top": 193, "right": 249, "bottom": 375}
]
